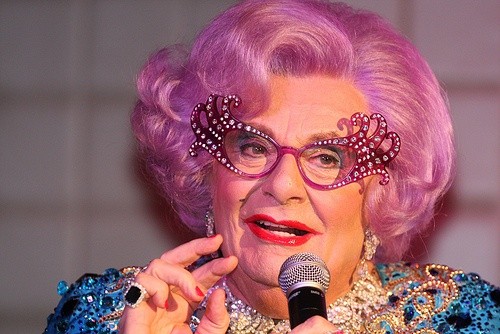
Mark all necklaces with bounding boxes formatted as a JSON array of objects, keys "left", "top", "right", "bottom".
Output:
[{"left": 201, "top": 277, "right": 390, "bottom": 334}]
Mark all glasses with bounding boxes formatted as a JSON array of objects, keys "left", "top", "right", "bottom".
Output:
[{"left": 188, "top": 94, "right": 401, "bottom": 190}]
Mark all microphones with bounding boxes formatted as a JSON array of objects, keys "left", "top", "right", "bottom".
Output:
[{"left": 277, "top": 252, "right": 331, "bottom": 333}]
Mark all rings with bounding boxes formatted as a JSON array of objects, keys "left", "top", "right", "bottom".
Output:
[{"left": 123, "top": 281, "right": 147, "bottom": 308}]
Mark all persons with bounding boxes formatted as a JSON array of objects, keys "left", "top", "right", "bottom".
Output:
[{"left": 40, "top": 0, "right": 500, "bottom": 334}]
